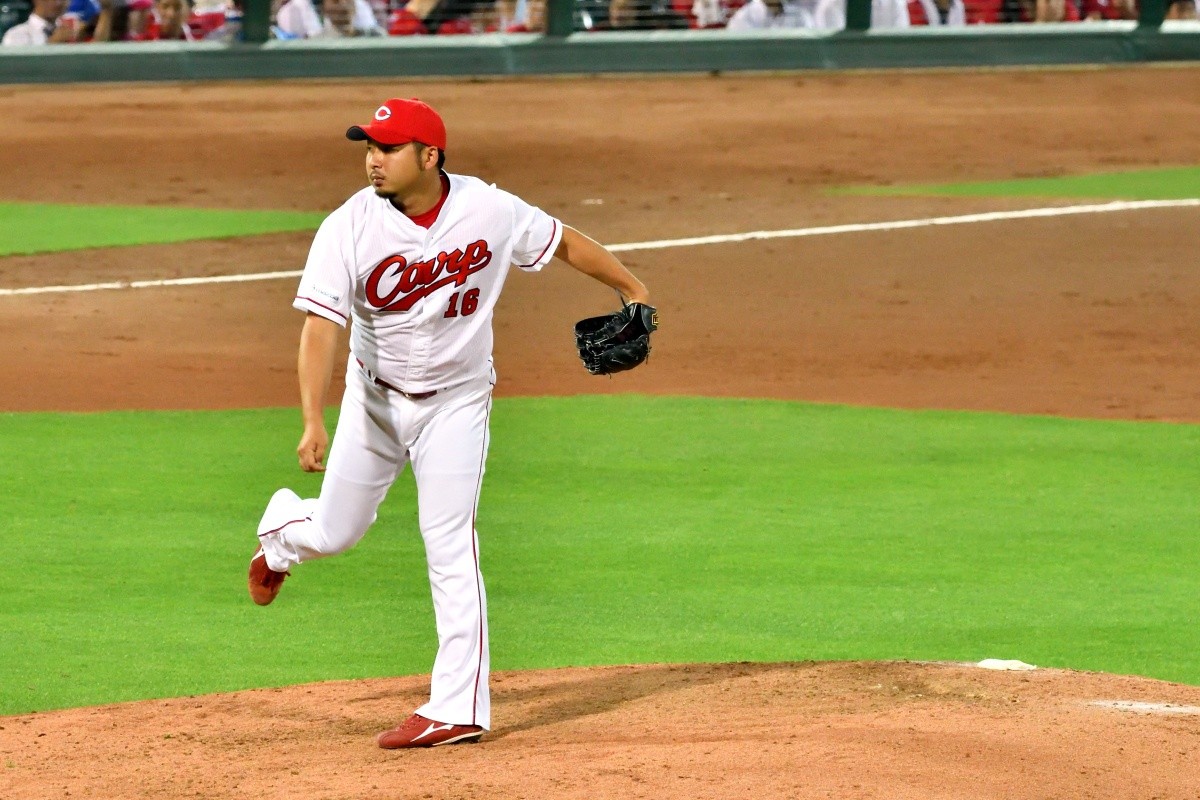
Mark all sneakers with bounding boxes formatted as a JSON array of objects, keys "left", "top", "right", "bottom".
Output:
[
  {"left": 378, "top": 714, "right": 484, "bottom": 749},
  {"left": 249, "top": 543, "right": 291, "bottom": 605}
]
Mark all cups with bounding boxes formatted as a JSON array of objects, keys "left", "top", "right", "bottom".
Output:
[{"left": 60, "top": 16, "right": 74, "bottom": 44}]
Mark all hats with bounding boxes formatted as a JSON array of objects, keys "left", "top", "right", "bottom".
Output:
[{"left": 346, "top": 98, "right": 446, "bottom": 152}]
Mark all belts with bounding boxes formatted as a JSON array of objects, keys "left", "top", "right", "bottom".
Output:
[{"left": 356, "top": 358, "right": 446, "bottom": 400}]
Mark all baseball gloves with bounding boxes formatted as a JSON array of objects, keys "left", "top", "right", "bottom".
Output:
[{"left": 574, "top": 301, "right": 660, "bottom": 375}]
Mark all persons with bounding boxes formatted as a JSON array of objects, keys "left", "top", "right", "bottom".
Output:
[
  {"left": 247, "top": 97, "right": 650, "bottom": 749},
  {"left": 0, "top": 0, "right": 1200, "bottom": 48}
]
